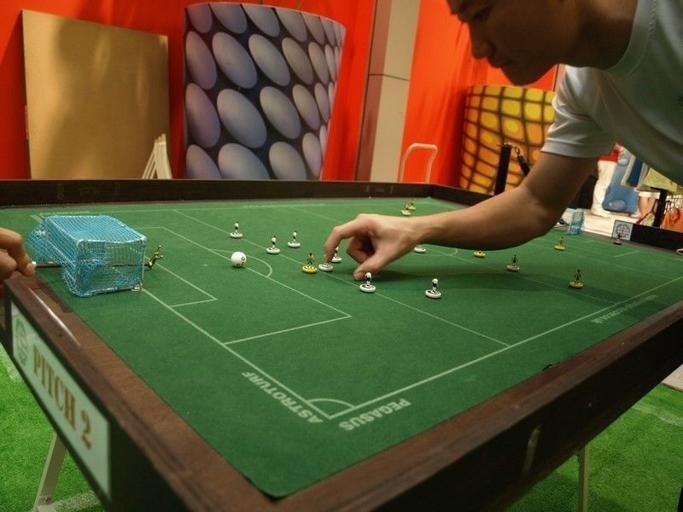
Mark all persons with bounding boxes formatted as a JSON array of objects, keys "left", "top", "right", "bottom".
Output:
[
  {"left": 291, "top": 230, "right": 297, "bottom": 243},
  {"left": 573, "top": 269, "right": 582, "bottom": 284},
  {"left": 271, "top": 235, "right": 277, "bottom": 250},
  {"left": 558, "top": 236, "right": 564, "bottom": 247},
  {"left": 616, "top": 233, "right": 622, "bottom": 241},
  {"left": 234, "top": 220, "right": 239, "bottom": 233},
  {"left": 306, "top": 253, "right": 316, "bottom": 268},
  {"left": 324, "top": 0, "right": 683, "bottom": 282},
  {"left": 0, "top": 227, "right": 37, "bottom": 282},
  {"left": 365, "top": 270, "right": 372, "bottom": 286},
  {"left": 431, "top": 276, "right": 439, "bottom": 293},
  {"left": 403, "top": 199, "right": 417, "bottom": 212},
  {"left": 512, "top": 254, "right": 518, "bottom": 268}
]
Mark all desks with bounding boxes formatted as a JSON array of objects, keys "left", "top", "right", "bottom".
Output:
[{"left": 0, "top": 178, "right": 681, "bottom": 510}]
